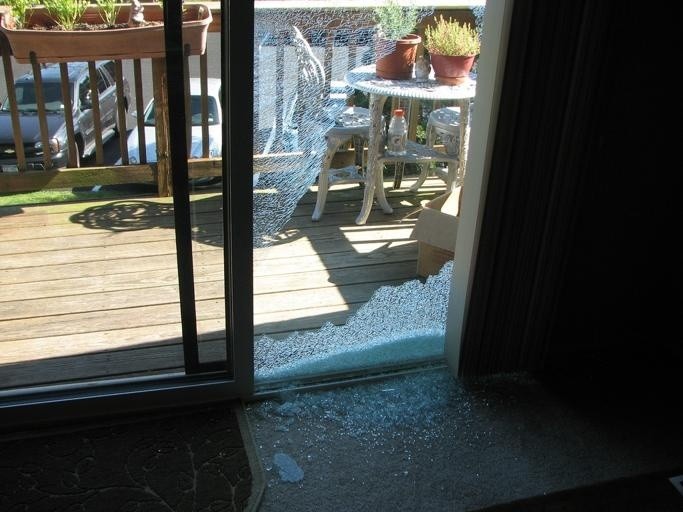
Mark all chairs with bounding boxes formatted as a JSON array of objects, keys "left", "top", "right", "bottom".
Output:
[
  {"left": 409, "top": 99, "right": 473, "bottom": 194},
  {"left": 46, "top": 86, "right": 57, "bottom": 98},
  {"left": 288, "top": 24, "right": 394, "bottom": 222}
]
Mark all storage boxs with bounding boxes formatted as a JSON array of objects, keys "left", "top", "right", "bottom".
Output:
[{"left": 398, "top": 184, "right": 460, "bottom": 279}]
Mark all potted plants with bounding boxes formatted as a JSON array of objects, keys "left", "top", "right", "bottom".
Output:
[
  {"left": 0, "top": 0, "right": 213, "bottom": 64},
  {"left": 370, "top": 0, "right": 422, "bottom": 80},
  {"left": 422, "top": 13, "right": 482, "bottom": 86}
]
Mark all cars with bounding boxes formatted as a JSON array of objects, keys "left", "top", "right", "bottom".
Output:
[{"left": 122, "top": 79, "right": 223, "bottom": 163}]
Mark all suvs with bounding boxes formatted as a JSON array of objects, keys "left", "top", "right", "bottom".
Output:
[
  {"left": 0, "top": 61, "right": 131, "bottom": 177},
  {"left": 278, "top": 79, "right": 347, "bottom": 158}
]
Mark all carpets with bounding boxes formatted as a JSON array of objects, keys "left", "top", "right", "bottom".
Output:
[{"left": 233, "top": 367, "right": 683, "bottom": 512}]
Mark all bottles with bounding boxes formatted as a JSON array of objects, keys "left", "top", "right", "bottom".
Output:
[{"left": 385, "top": 108, "right": 409, "bottom": 156}]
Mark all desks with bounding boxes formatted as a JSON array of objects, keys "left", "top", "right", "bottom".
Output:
[{"left": 345, "top": 61, "right": 477, "bottom": 226}]
